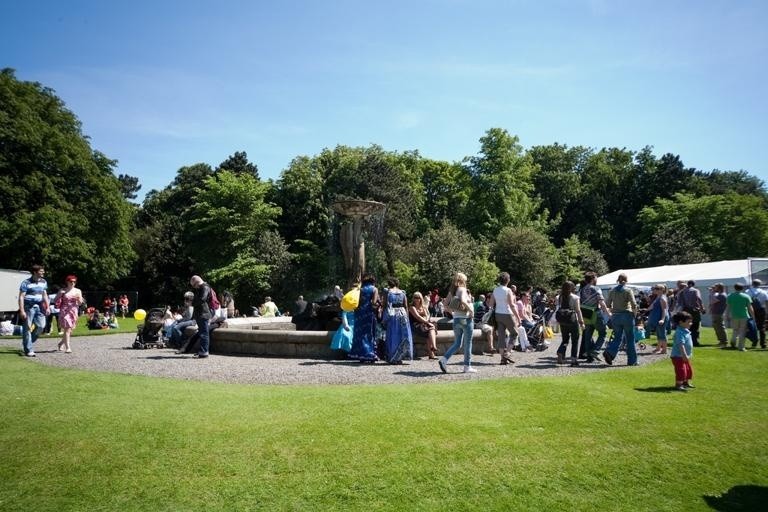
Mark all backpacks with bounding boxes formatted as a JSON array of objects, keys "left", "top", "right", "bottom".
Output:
[
  {"left": 750, "top": 288, "right": 765, "bottom": 319},
  {"left": 199, "top": 283, "right": 220, "bottom": 309}
]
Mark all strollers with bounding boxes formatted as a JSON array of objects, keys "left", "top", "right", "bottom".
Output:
[
  {"left": 515, "top": 306, "right": 557, "bottom": 351},
  {"left": 607, "top": 317, "right": 646, "bottom": 351},
  {"left": 131, "top": 306, "right": 170, "bottom": 351}
]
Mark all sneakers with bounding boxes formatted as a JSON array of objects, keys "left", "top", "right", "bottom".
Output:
[
  {"left": 429, "top": 356, "right": 439, "bottom": 360},
  {"left": 431, "top": 347, "right": 440, "bottom": 352},
  {"left": 675, "top": 383, "right": 688, "bottom": 391},
  {"left": 25, "top": 344, "right": 72, "bottom": 357},
  {"left": 694, "top": 339, "right": 766, "bottom": 352},
  {"left": 485, "top": 341, "right": 549, "bottom": 365},
  {"left": 558, "top": 350, "right": 640, "bottom": 367},
  {"left": 684, "top": 382, "right": 696, "bottom": 388}
]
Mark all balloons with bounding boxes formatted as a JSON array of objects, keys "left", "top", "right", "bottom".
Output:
[{"left": 133, "top": 309, "right": 146, "bottom": 321}]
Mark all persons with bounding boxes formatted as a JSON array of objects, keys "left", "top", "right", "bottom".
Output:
[
  {"left": 0, "top": 264, "right": 130, "bottom": 357},
  {"left": 166, "top": 275, "right": 308, "bottom": 358},
  {"left": 329, "top": 271, "right": 768, "bottom": 389}
]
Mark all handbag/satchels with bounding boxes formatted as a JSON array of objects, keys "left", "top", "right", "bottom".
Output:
[
  {"left": 580, "top": 305, "right": 597, "bottom": 325},
  {"left": 486, "top": 300, "right": 496, "bottom": 326},
  {"left": 556, "top": 308, "right": 576, "bottom": 325},
  {"left": 448, "top": 286, "right": 467, "bottom": 312},
  {"left": 54, "top": 288, "right": 65, "bottom": 309},
  {"left": 340, "top": 282, "right": 362, "bottom": 312},
  {"left": 543, "top": 316, "right": 553, "bottom": 339},
  {"left": 381, "top": 288, "right": 395, "bottom": 325}
]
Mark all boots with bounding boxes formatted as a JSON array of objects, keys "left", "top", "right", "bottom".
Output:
[
  {"left": 464, "top": 365, "right": 477, "bottom": 373},
  {"left": 438, "top": 356, "right": 448, "bottom": 374}
]
